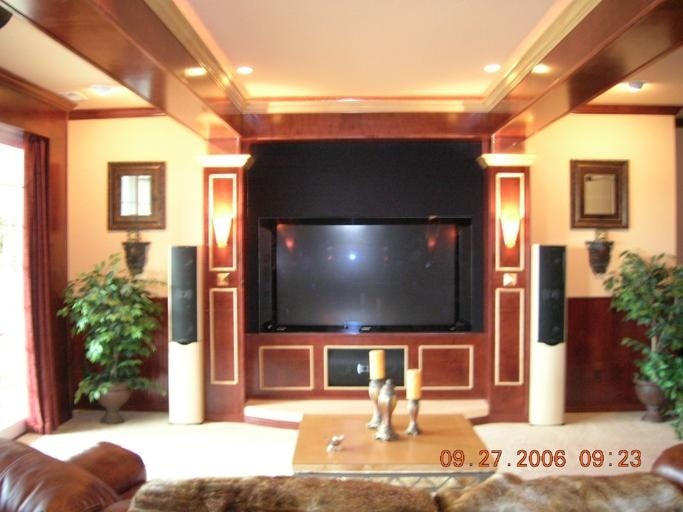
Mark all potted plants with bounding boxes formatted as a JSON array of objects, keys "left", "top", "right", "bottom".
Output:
[
  {"left": 55, "top": 250, "right": 167, "bottom": 424},
  {"left": 601, "top": 247, "right": 683, "bottom": 423}
]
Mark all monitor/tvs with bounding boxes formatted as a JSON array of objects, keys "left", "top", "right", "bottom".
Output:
[{"left": 272, "top": 222, "right": 459, "bottom": 327}]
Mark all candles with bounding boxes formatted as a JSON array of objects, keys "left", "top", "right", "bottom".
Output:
[
  {"left": 369, "top": 349, "right": 386, "bottom": 379},
  {"left": 405, "top": 368, "right": 422, "bottom": 399}
]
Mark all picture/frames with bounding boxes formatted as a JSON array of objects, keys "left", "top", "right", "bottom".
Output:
[
  {"left": 568, "top": 158, "right": 630, "bottom": 230},
  {"left": 107, "top": 161, "right": 166, "bottom": 231}
]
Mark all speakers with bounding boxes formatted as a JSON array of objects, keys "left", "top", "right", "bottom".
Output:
[
  {"left": 529, "top": 244, "right": 567, "bottom": 426},
  {"left": 167, "top": 244, "right": 205, "bottom": 425}
]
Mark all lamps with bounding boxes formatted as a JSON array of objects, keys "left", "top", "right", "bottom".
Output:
[
  {"left": 501, "top": 205, "right": 522, "bottom": 248},
  {"left": 213, "top": 207, "right": 232, "bottom": 249}
]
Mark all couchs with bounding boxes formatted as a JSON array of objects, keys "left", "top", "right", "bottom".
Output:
[{"left": 0, "top": 438, "right": 683, "bottom": 512}]
